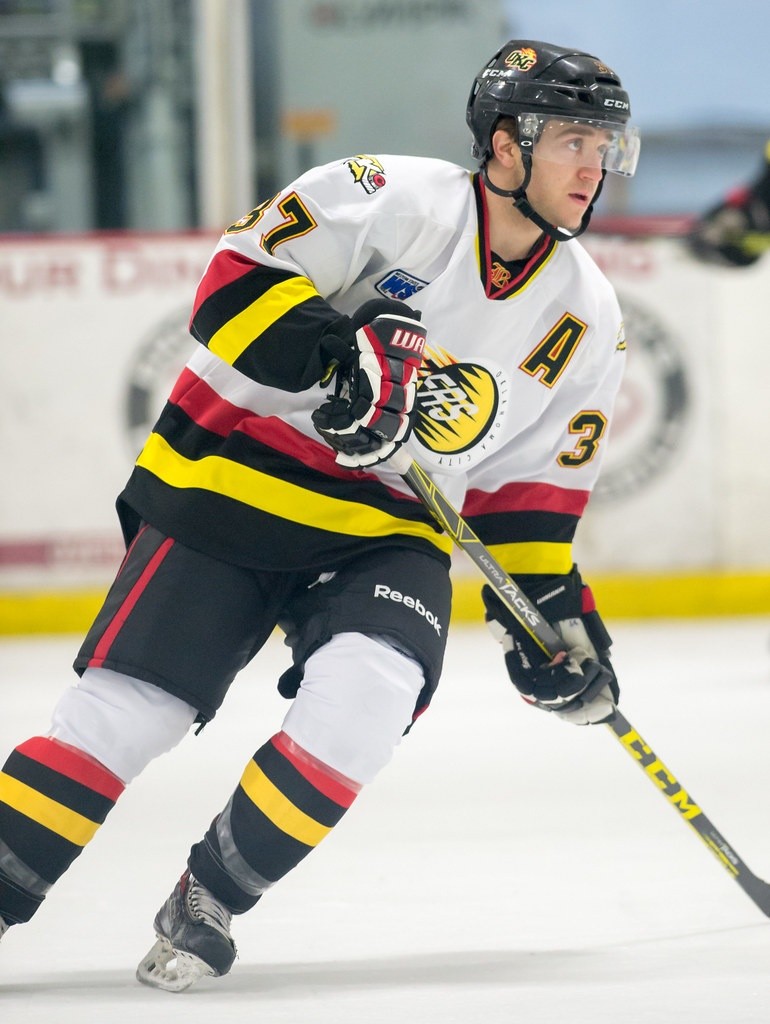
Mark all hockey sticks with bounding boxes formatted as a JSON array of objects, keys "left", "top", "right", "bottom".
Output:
[{"left": 335, "top": 372, "right": 770, "bottom": 920}]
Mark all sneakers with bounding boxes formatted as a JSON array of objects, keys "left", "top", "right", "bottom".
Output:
[{"left": 137, "top": 865, "right": 236, "bottom": 992}]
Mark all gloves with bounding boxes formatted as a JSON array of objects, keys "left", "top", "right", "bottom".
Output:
[
  {"left": 311, "top": 301, "right": 427, "bottom": 469},
  {"left": 483, "top": 568, "right": 621, "bottom": 729}
]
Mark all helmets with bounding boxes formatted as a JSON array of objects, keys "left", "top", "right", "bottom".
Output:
[{"left": 465, "top": 39, "right": 632, "bottom": 158}]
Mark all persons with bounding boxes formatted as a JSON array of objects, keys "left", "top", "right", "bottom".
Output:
[{"left": 0, "top": 34, "right": 623, "bottom": 992}]
